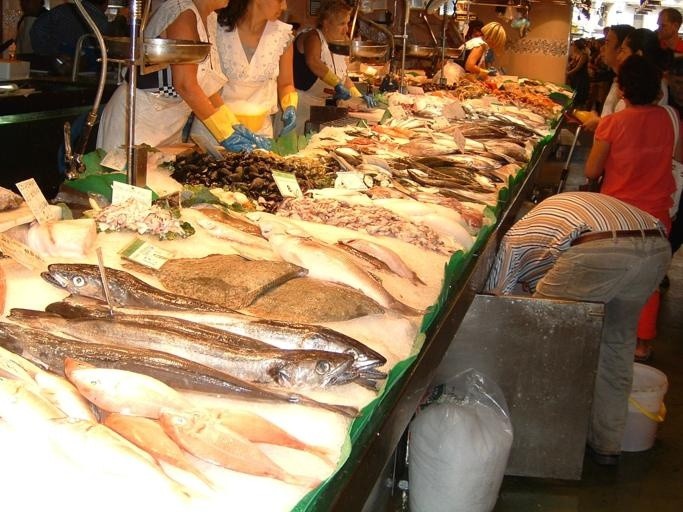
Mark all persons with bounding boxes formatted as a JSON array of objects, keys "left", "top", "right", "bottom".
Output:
[
  {"left": 565, "top": 9, "right": 681, "bottom": 360},
  {"left": 272, "top": 1, "right": 381, "bottom": 144},
  {"left": 11, "top": 0, "right": 51, "bottom": 62},
  {"left": 93, "top": 0, "right": 272, "bottom": 174},
  {"left": 480, "top": 191, "right": 673, "bottom": 467},
  {"left": 430, "top": 21, "right": 507, "bottom": 86},
  {"left": 185, "top": 1, "right": 299, "bottom": 149},
  {"left": 464, "top": 19, "right": 494, "bottom": 70},
  {"left": 28, "top": 1, "right": 114, "bottom": 60}
]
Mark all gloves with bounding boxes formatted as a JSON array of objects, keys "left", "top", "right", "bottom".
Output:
[
  {"left": 278, "top": 92, "right": 299, "bottom": 135},
  {"left": 324, "top": 69, "right": 348, "bottom": 99},
  {"left": 480, "top": 67, "right": 497, "bottom": 76},
  {"left": 202, "top": 105, "right": 271, "bottom": 154},
  {"left": 349, "top": 87, "right": 377, "bottom": 109}
]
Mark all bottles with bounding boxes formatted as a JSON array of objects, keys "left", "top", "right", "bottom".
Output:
[{"left": 571, "top": 107, "right": 602, "bottom": 133}]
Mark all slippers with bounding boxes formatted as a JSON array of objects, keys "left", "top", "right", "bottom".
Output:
[{"left": 634, "top": 343, "right": 655, "bottom": 359}]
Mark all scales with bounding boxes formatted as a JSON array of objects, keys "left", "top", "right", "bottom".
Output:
[
  {"left": 64, "top": 0, "right": 212, "bottom": 188},
  {"left": 327, "top": 0, "right": 465, "bottom": 96}
]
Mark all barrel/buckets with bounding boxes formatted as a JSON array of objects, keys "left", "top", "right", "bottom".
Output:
[{"left": 621, "top": 363, "right": 669, "bottom": 452}]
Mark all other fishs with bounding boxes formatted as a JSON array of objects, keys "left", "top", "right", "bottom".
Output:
[{"left": 0, "top": 202, "right": 433, "bottom": 500}]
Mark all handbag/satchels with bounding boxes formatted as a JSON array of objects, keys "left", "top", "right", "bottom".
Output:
[{"left": 669, "top": 159, "right": 682, "bottom": 217}]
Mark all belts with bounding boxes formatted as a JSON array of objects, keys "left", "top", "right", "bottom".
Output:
[{"left": 572, "top": 229, "right": 666, "bottom": 244}]
